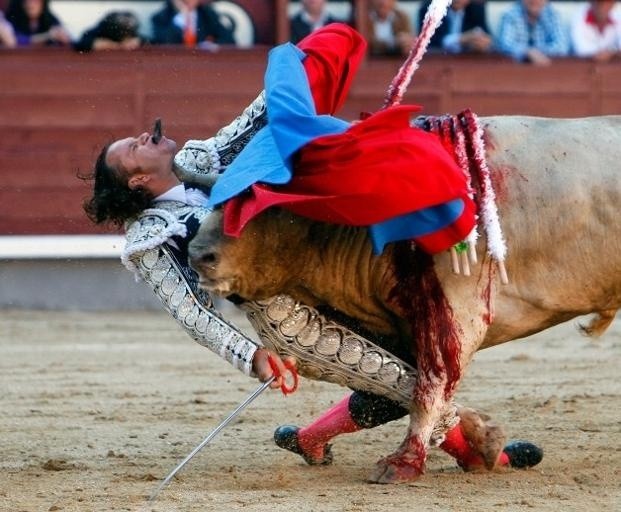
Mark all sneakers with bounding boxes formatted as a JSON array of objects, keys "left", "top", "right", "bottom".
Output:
[
  {"left": 459, "top": 442, "right": 543, "bottom": 472},
  {"left": 274, "top": 425, "right": 332, "bottom": 465}
]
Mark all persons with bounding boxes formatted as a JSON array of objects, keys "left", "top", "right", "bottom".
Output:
[
  {"left": 286, "top": 1, "right": 621, "bottom": 68},
  {"left": 0, "top": 1, "right": 235, "bottom": 51},
  {"left": 74, "top": 89, "right": 543, "bottom": 470}
]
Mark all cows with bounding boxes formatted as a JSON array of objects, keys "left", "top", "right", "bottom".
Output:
[{"left": 150, "top": 115, "right": 621, "bottom": 485}]
